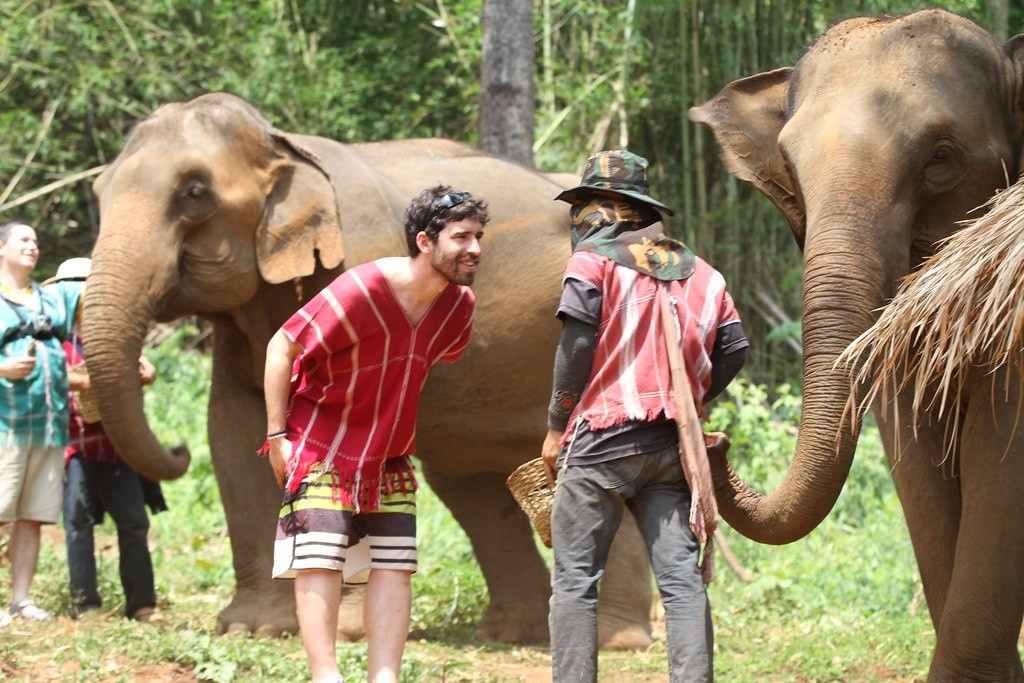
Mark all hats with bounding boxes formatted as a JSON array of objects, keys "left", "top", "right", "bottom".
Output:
[
  {"left": 40, "top": 257, "right": 91, "bottom": 287},
  {"left": 553, "top": 150, "right": 675, "bottom": 217}
]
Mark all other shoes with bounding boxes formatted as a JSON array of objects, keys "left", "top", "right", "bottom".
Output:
[
  {"left": 134, "top": 607, "right": 164, "bottom": 623},
  {"left": 74, "top": 606, "right": 106, "bottom": 620}
]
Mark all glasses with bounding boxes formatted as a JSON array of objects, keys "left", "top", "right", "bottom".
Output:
[{"left": 422, "top": 192, "right": 472, "bottom": 231}]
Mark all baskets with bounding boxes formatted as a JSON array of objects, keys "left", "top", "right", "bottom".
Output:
[
  {"left": 71, "top": 362, "right": 101, "bottom": 423},
  {"left": 506, "top": 457, "right": 557, "bottom": 549}
]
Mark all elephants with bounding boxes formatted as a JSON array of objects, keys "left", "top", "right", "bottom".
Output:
[
  {"left": 689, "top": 9, "right": 1024, "bottom": 683},
  {"left": 80, "top": 92, "right": 653, "bottom": 651}
]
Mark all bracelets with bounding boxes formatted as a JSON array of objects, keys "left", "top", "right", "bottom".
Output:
[{"left": 267, "top": 430, "right": 288, "bottom": 441}]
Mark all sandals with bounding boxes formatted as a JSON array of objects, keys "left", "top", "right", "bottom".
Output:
[{"left": 9, "top": 600, "right": 49, "bottom": 622}]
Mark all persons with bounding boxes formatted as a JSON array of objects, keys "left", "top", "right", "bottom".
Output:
[
  {"left": 0, "top": 219, "right": 87, "bottom": 631},
  {"left": 256, "top": 185, "right": 492, "bottom": 683},
  {"left": 40, "top": 257, "right": 170, "bottom": 625},
  {"left": 540, "top": 149, "right": 751, "bottom": 682}
]
